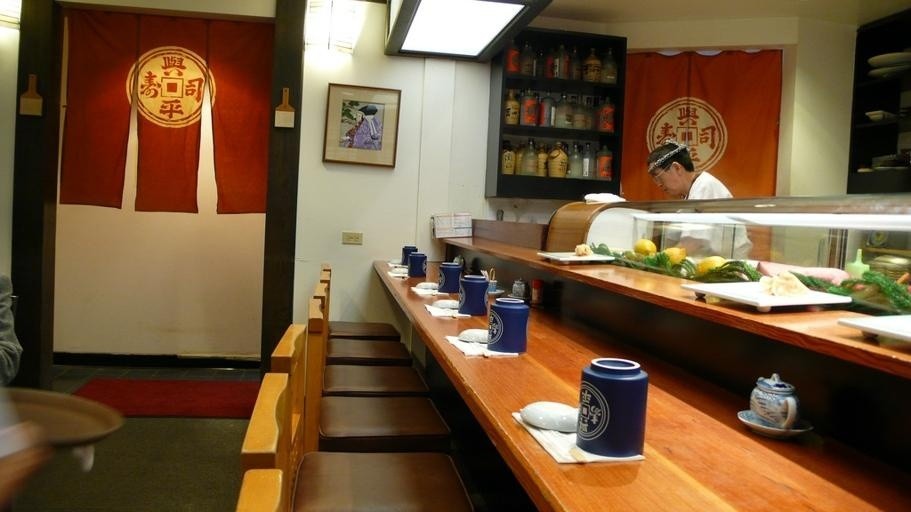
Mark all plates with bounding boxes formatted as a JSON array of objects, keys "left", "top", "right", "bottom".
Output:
[
  {"left": 681, "top": 277, "right": 855, "bottom": 312},
  {"left": 856, "top": 153, "right": 909, "bottom": 173},
  {"left": 866, "top": 111, "right": 894, "bottom": 122},
  {"left": 737, "top": 409, "right": 814, "bottom": 438},
  {"left": 869, "top": 51, "right": 911, "bottom": 77},
  {"left": 537, "top": 249, "right": 617, "bottom": 265},
  {"left": 508, "top": 293, "right": 527, "bottom": 300},
  {"left": 838, "top": 312, "right": 911, "bottom": 345},
  {"left": 490, "top": 290, "right": 505, "bottom": 296}
]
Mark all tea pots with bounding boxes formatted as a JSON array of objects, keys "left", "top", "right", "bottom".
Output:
[
  {"left": 513, "top": 276, "right": 530, "bottom": 296},
  {"left": 749, "top": 373, "right": 801, "bottom": 428}
]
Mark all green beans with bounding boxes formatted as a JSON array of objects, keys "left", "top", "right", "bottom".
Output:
[
  {"left": 825, "top": 269, "right": 911, "bottom": 311},
  {"left": 648, "top": 252, "right": 698, "bottom": 278},
  {"left": 789, "top": 271, "right": 836, "bottom": 290},
  {"left": 589, "top": 241, "right": 646, "bottom": 271},
  {"left": 694, "top": 260, "right": 763, "bottom": 282}
]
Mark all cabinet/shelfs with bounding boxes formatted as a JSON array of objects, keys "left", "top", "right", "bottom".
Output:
[
  {"left": 844, "top": 6, "right": 910, "bottom": 196},
  {"left": 486, "top": 26, "right": 629, "bottom": 199},
  {"left": 535, "top": 195, "right": 911, "bottom": 324}
]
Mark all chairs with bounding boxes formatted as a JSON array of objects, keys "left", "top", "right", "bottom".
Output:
[{"left": 229, "top": 256, "right": 483, "bottom": 512}]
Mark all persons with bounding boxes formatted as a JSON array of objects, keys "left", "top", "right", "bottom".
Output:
[
  {"left": 646, "top": 142, "right": 753, "bottom": 260},
  {"left": 0, "top": 275, "right": 24, "bottom": 387},
  {"left": 0, "top": 380, "right": 49, "bottom": 512}
]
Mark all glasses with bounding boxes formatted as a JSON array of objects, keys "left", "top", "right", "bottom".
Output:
[{"left": 649, "top": 163, "right": 673, "bottom": 185}]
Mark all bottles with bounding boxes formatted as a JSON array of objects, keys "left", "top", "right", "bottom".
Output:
[
  {"left": 504, "top": 88, "right": 616, "bottom": 132},
  {"left": 532, "top": 279, "right": 544, "bottom": 304},
  {"left": 506, "top": 38, "right": 620, "bottom": 84},
  {"left": 848, "top": 247, "right": 872, "bottom": 282},
  {"left": 502, "top": 138, "right": 613, "bottom": 183}
]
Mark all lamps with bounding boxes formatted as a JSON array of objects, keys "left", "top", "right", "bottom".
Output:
[{"left": 365, "top": 0, "right": 552, "bottom": 64}]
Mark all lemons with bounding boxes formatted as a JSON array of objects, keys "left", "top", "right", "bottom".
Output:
[{"left": 634, "top": 238, "right": 657, "bottom": 255}]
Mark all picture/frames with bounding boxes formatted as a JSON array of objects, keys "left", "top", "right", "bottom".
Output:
[{"left": 322, "top": 81, "right": 403, "bottom": 169}]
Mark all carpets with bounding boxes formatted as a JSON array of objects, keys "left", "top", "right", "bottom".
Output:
[{"left": 68, "top": 374, "right": 263, "bottom": 419}]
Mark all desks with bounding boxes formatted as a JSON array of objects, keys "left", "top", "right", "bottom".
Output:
[{"left": 1, "top": 374, "right": 124, "bottom": 510}]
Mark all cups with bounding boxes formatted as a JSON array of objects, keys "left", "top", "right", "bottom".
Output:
[
  {"left": 486, "top": 298, "right": 530, "bottom": 353},
  {"left": 458, "top": 275, "right": 490, "bottom": 316},
  {"left": 401, "top": 245, "right": 419, "bottom": 265},
  {"left": 438, "top": 262, "right": 462, "bottom": 293},
  {"left": 488, "top": 280, "right": 497, "bottom": 292},
  {"left": 408, "top": 252, "right": 428, "bottom": 277},
  {"left": 576, "top": 355, "right": 651, "bottom": 461}
]
